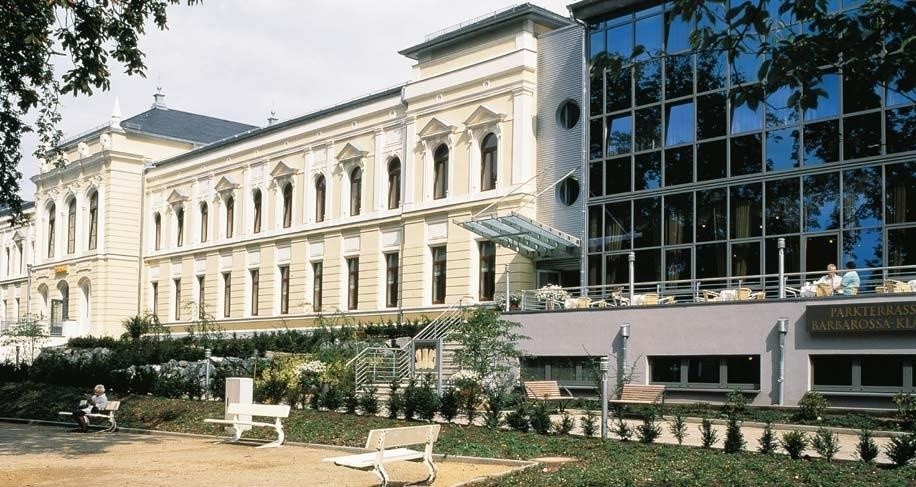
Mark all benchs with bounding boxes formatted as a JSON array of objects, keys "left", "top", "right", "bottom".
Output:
[
  {"left": 202, "top": 401, "right": 292, "bottom": 448},
  {"left": 322, "top": 421, "right": 443, "bottom": 486},
  {"left": 59, "top": 397, "right": 121, "bottom": 436},
  {"left": 517, "top": 379, "right": 665, "bottom": 420}
]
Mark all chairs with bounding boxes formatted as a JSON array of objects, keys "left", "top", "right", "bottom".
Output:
[
  {"left": 875, "top": 279, "right": 916, "bottom": 294},
  {"left": 695, "top": 288, "right": 766, "bottom": 305},
  {"left": 565, "top": 293, "right": 678, "bottom": 308},
  {"left": 800, "top": 281, "right": 859, "bottom": 296}
]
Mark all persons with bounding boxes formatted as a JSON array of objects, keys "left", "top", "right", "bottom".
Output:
[
  {"left": 72, "top": 384, "right": 107, "bottom": 432},
  {"left": 817, "top": 262, "right": 842, "bottom": 290},
  {"left": 833, "top": 261, "right": 861, "bottom": 295}
]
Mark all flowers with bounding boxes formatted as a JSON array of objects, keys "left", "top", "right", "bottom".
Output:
[{"left": 535, "top": 282, "right": 570, "bottom": 301}]
